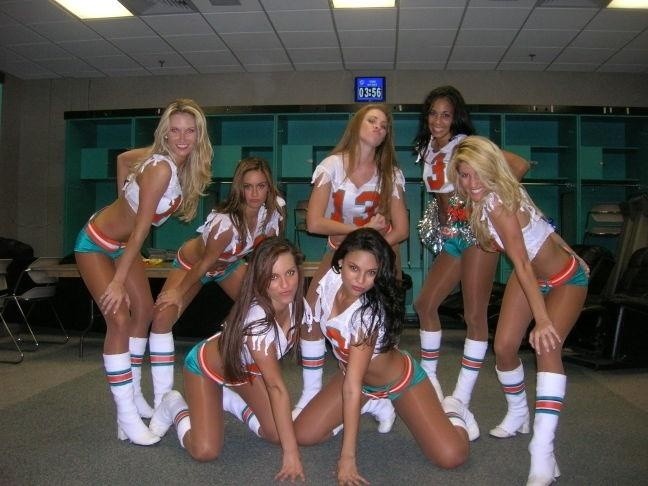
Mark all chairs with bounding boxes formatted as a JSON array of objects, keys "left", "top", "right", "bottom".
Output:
[
  {"left": 0, "top": 257, "right": 38, "bottom": 362},
  {"left": 13, "top": 258, "right": 71, "bottom": 344},
  {"left": 561, "top": 244, "right": 615, "bottom": 353},
  {"left": 561, "top": 247, "right": 648, "bottom": 369}
]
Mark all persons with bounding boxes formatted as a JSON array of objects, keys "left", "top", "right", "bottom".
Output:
[
  {"left": 149, "top": 156, "right": 287, "bottom": 411},
  {"left": 289, "top": 104, "right": 409, "bottom": 421},
  {"left": 413, "top": 85, "right": 531, "bottom": 407},
  {"left": 294, "top": 227, "right": 479, "bottom": 486},
  {"left": 75, "top": 99, "right": 214, "bottom": 446},
  {"left": 149, "top": 235, "right": 306, "bottom": 485},
  {"left": 433, "top": 135, "right": 591, "bottom": 486}
]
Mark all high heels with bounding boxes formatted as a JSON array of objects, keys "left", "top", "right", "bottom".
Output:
[
  {"left": 115, "top": 419, "right": 162, "bottom": 446},
  {"left": 133, "top": 396, "right": 158, "bottom": 419},
  {"left": 376, "top": 399, "right": 397, "bottom": 434},
  {"left": 524, "top": 454, "right": 561, "bottom": 485},
  {"left": 489, "top": 412, "right": 530, "bottom": 437},
  {"left": 292, "top": 393, "right": 314, "bottom": 422},
  {"left": 443, "top": 396, "right": 482, "bottom": 441},
  {"left": 149, "top": 390, "right": 180, "bottom": 438}
]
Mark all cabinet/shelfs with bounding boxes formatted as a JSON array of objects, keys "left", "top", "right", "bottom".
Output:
[{"left": 61, "top": 104, "right": 644, "bottom": 316}]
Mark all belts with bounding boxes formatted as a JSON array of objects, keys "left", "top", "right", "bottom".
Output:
[
  {"left": 325, "top": 238, "right": 339, "bottom": 255},
  {"left": 346, "top": 353, "right": 414, "bottom": 400},
  {"left": 175, "top": 248, "right": 246, "bottom": 279},
  {"left": 534, "top": 254, "right": 579, "bottom": 291},
  {"left": 86, "top": 210, "right": 128, "bottom": 250},
  {"left": 198, "top": 335, "right": 252, "bottom": 389}
]
